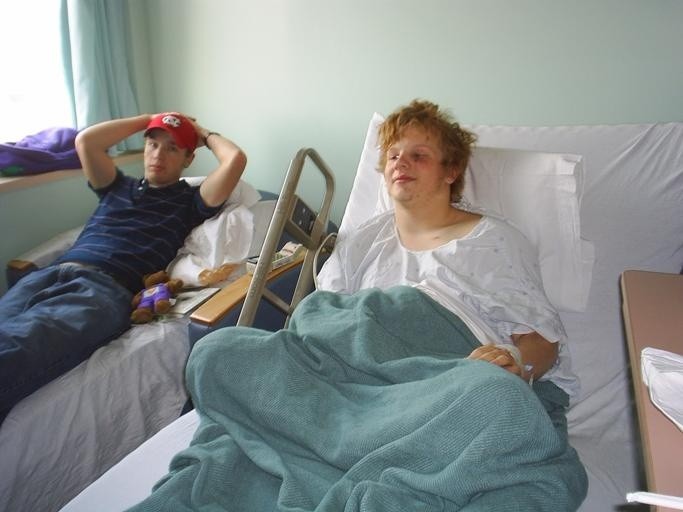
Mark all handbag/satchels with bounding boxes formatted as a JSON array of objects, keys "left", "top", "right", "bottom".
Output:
[{"left": 0, "top": 125, "right": 83, "bottom": 175}]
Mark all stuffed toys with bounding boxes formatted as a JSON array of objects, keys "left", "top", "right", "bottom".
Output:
[{"left": 130, "top": 268, "right": 184, "bottom": 324}]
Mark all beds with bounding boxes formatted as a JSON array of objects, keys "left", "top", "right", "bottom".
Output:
[
  {"left": 0, "top": 168, "right": 337, "bottom": 512},
  {"left": 54, "top": 112, "right": 683, "bottom": 512}
]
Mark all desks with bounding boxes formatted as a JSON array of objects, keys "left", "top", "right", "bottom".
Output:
[{"left": 618, "top": 270, "right": 683, "bottom": 512}]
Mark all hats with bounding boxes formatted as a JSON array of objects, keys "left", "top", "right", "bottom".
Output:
[{"left": 144, "top": 113, "right": 198, "bottom": 151}]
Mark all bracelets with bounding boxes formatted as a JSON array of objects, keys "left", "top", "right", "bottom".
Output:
[{"left": 203, "top": 131, "right": 220, "bottom": 148}]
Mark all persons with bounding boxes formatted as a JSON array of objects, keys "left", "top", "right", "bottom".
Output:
[
  {"left": 0, "top": 112, "right": 247, "bottom": 431},
  {"left": 315, "top": 98, "right": 569, "bottom": 385}
]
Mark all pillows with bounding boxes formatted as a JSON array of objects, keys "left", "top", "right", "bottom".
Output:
[
  {"left": 377, "top": 149, "right": 594, "bottom": 317},
  {"left": 180, "top": 172, "right": 263, "bottom": 208}
]
